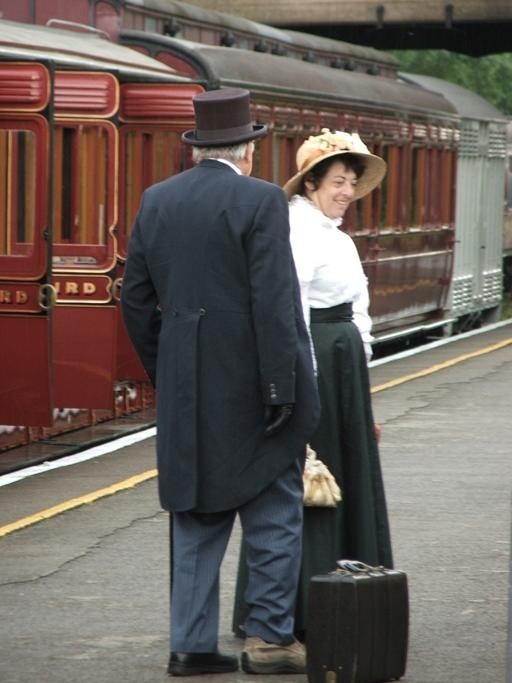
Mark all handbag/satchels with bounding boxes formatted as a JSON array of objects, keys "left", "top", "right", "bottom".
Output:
[{"left": 302, "top": 443, "right": 344, "bottom": 511}]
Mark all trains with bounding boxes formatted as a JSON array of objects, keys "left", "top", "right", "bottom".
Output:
[{"left": 1, "top": 1, "right": 512, "bottom": 460}]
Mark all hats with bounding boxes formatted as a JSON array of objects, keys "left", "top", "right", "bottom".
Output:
[
  {"left": 281, "top": 127, "right": 391, "bottom": 205},
  {"left": 178, "top": 85, "right": 270, "bottom": 150}
]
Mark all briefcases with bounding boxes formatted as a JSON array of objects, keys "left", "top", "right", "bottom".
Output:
[{"left": 303, "top": 557, "right": 411, "bottom": 682}]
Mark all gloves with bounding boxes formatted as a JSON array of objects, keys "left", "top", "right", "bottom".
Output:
[{"left": 262, "top": 401, "right": 296, "bottom": 436}]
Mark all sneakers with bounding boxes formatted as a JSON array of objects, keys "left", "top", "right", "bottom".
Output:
[{"left": 239, "top": 632, "right": 309, "bottom": 675}]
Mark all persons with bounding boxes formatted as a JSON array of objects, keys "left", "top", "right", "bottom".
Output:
[
  {"left": 119, "top": 85, "right": 323, "bottom": 680},
  {"left": 230, "top": 127, "right": 397, "bottom": 640}
]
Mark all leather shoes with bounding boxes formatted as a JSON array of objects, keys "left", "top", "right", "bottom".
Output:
[{"left": 165, "top": 640, "right": 241, "bottom": 677}]
